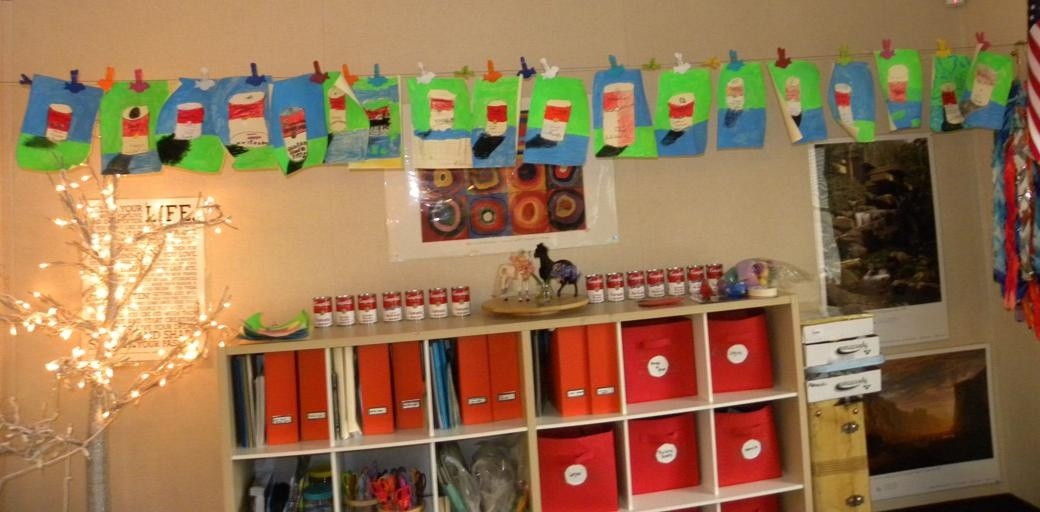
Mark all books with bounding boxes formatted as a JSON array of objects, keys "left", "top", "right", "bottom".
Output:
[
  {"left": 253, "top": 374, "right": 268, "bottom": 452},
  {"left": 438, "top": 495, "right": 452, "bottom": 512},
  {"left": 429, "top": 340, "right": 462, "bottom": 432},
  {"left": 332, "top": 346, "right": 350, "bottom": 439},
  {"left": 240, "top": 353, "right": 256, "bottom": 450},
  {"left": 436, "top": 458, "right": 467, "bottom": 512},
  {"left": 330, "top": 372, "right": 340, "bottom": 442},
  {"left": 345, "top": 344, "right": 366, "bottom": 439}
]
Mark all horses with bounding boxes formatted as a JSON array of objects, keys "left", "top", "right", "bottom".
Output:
[
  {"left": 533, "top": 242, "right": 581, "bottom": 298},
  {"left": 491, "top": 249, "right": 534, "bottom": 302}
]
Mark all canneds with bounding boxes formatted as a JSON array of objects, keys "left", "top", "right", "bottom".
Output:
[
  {"left": 313, "top": 286, "right": 471, "bottom": 328},
  {"left": 584, "top": 262, "right": 723, "bottom": 304}
]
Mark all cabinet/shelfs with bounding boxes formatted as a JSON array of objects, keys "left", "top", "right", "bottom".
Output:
[{"left": 216, "top": 286, "right": 815, "bottom": 511}]
